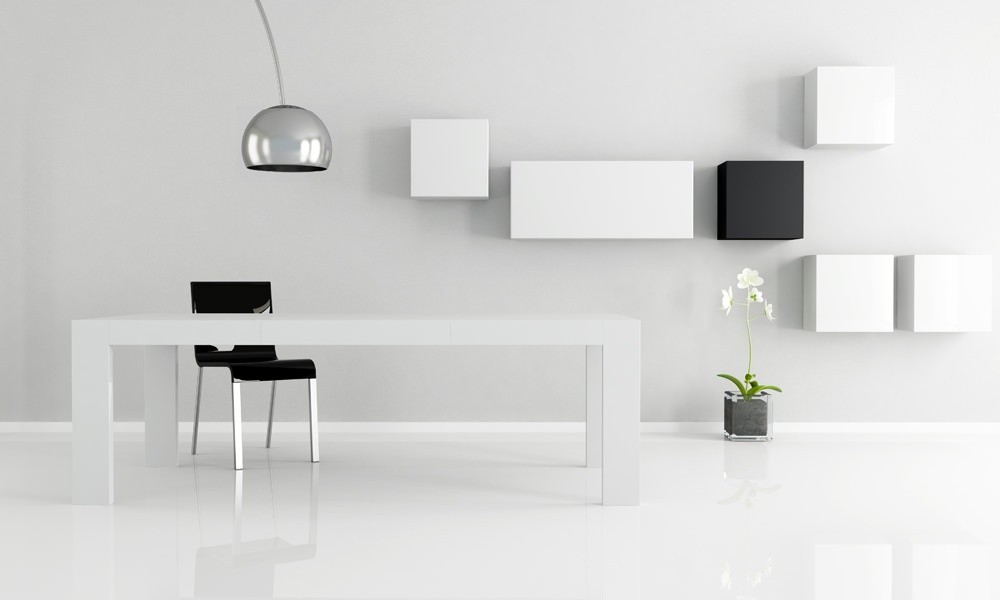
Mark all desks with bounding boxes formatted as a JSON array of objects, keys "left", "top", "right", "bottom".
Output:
[{"left": 71, "top": 319, "right": 641, "bottom": 508}]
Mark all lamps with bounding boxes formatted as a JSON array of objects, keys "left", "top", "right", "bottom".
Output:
[{"left": 240, "top": 0, "right": 331, "bottom": 172}]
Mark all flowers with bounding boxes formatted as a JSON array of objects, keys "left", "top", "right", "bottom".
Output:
[{"left": 718, "top": 267, "right": 782, "bottom": 402}]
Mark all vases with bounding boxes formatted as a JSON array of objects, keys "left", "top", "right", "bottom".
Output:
[{"left": 723, "top": 390, "right": 773, "bottom": 443}]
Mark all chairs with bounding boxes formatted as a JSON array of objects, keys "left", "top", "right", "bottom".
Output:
[{"left": 190, "top": 282, "right": 320, "bottom": 472}]
